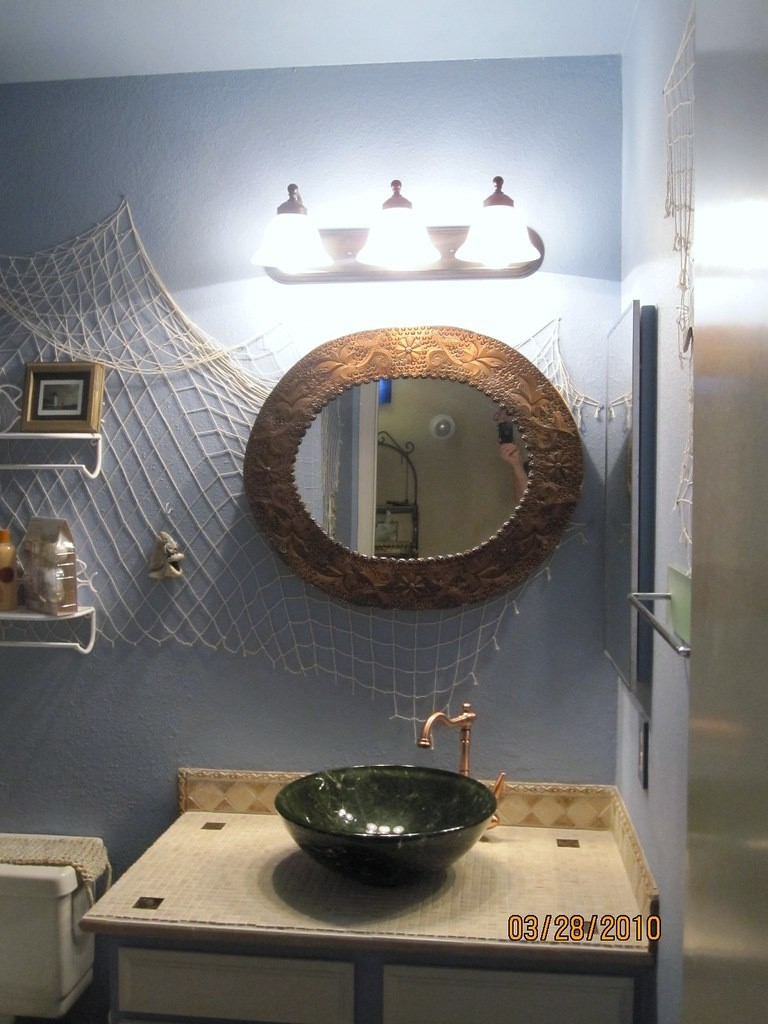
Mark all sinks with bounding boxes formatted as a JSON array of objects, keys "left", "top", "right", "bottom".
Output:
[{"left": 274, "top": 764, "right": 497, "bottom": 889}]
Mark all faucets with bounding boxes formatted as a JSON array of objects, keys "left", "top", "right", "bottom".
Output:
[{"left": 418, "top": 703, "right": 476, "bottom": 777}]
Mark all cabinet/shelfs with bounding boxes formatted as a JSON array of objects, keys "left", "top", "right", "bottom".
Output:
[
  {"left": 375, "top": 430, "right": 420, "bottom": 557},
  {"left": 0, "top": 433, "right": 104, "bottom": 656},
  {"left": 84, "top": 766, "right": 658, "bottom": 1024}
]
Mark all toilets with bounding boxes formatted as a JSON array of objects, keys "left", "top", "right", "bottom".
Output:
[{"left": 0, "top": 832, "right": 103, "bottom": 1024}]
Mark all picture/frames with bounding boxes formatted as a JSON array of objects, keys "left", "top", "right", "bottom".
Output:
[
  {"left": 19, "top": 360, "right": 104, "bottom": 431},
  {"left": 599, "top": 300, "right": 642, "bottom": 694}
]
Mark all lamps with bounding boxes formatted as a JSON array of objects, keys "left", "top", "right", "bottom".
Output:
[{"left": 255, "top": 175, "right": 547, "bottom": 283}]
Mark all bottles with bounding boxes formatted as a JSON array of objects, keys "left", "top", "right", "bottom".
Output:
[{"left": 0, "top": 528, "right": 18, "bottom": 611}]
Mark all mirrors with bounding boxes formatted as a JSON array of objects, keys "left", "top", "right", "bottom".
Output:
[{"left": 245, "top": 325, "right": 587, "bottom": 608}]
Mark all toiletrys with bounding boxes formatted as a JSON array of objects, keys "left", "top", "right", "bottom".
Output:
[{"left": 0, "top": 530, "right": 17, "bottom": 611}]
